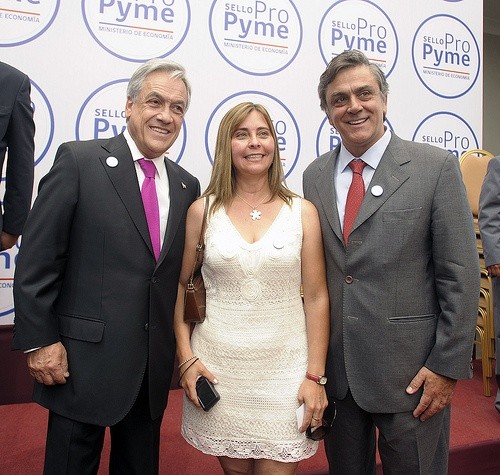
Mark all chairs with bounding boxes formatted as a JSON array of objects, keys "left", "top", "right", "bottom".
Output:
[{"left": 458, "top": 148, "right": 496, "bottom": 398}]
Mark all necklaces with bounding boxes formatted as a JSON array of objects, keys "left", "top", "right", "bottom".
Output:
[{"left": 235, "top": 190, "right": 270, "bottom": 221}]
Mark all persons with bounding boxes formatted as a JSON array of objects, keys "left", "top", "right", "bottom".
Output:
[
  {"left": 479, "top": 155, "right": 500, "bottom": 408},
  {"left": 13, "top": 60, "right": 201, "bottom": 475},
  {"left": 303, "top": 50, "right": 480, "bottom": 475},
  {"left": 0, "top": 60, "right": 36, "bottom": 251},
  {"left": 172, "top": 102, "right": 330, "bottom": 475}
]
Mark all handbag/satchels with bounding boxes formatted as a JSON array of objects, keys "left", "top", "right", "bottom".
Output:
[{"left": 182, "top": 195, "right": 209, "bottom": 324}]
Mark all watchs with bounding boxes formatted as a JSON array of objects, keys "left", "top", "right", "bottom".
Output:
[{"left": 304, "top": 371, "right": 327, "bottom": 386}]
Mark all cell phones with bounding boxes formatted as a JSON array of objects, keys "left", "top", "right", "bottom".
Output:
[{"left": 196, "top": 375, "right": 220, "bottom": 412}]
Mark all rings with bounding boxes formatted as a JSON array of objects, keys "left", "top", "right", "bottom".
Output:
[{"left": 312, "top": 418, "right": 320, "bottom": 421}]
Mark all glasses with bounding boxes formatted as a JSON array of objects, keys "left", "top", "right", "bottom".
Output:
[{"left": 306, "top": 401, "right": 336, "bottom": 441}]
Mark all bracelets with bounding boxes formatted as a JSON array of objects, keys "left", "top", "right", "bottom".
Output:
[{"left": 178, "top": 355, "right": 198, "bottom": 385}]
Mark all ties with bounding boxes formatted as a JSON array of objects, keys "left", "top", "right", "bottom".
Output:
[
  {"left": 343, "top": 158, "right": 368, "bottom": 247},
  {"left": 136, "top": 158, "right": 160, "bottom": 263}
]
